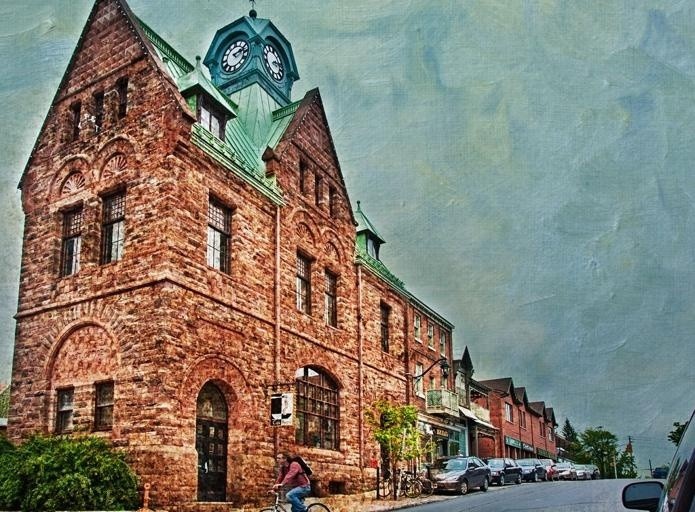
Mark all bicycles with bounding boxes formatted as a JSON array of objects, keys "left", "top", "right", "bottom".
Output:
[
  {"left": 376, "top": 471, "right": 435, "bottom": 500},
  {"left": 258, "top": 486, "right": 331, "bottom": 512}
]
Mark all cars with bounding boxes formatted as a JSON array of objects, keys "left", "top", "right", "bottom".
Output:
[
  {"left": 197, "top": 462, "right": 225, "bottom": 500},
  {"left": 623, "top": 407, "right": 695, "bottom": 512},
  {"left": 424, "top": 455, "right": 493, "bottom": 495},
  {"left": 652, "top": 467, "right": 669, "bottom": 479},
  {"left": 514, "top": 458, "right": 601, "bottom": 483},
  {"left": 481, "top": 456, "right": 523, "bottom": 487}
]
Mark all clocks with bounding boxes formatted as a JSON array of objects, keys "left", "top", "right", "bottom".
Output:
[
  {"left": 263, "top": 43, "right": 285, "bottom": 81},
  {"left": 220, "top": 39, "right": 249, "bottom": 73}
]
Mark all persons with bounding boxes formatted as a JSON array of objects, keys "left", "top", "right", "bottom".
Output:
[{"left": 265, "top": 451, "right": 313, "bottom": 512}]
